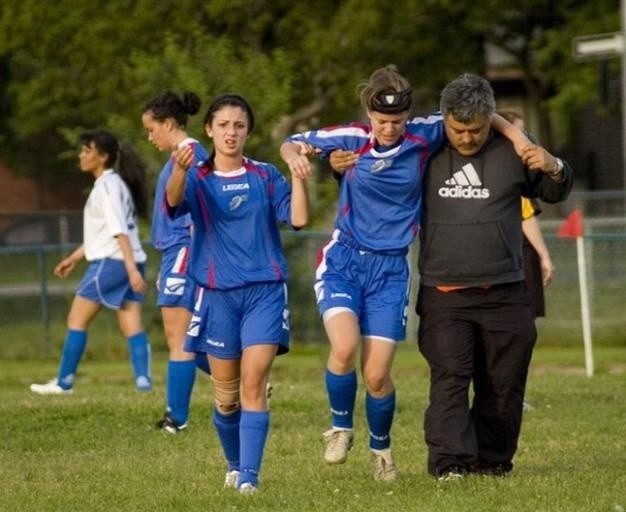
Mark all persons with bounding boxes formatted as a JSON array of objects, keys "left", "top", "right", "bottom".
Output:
[
  {"left": 327, "top": 72, "right": 574, "bottom": 484},
  {"left": 29, "top": 130, "right": 152, "bottom": 395},
  {"left": 495, "top": 105, "right": 554, "bottom": 415},
  {"left": 141, "top": 86, "right": 275, "bottom": 433},
  {"left": 279, "top": 62, "right": 535, "bottom": 483},
  {"left": 162, "top": 94, "right": 322, "bottom": 494}
]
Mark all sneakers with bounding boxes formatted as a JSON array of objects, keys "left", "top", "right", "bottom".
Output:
[
  {"left": 155, "top": 413, "right": 189, "bottom": 436},
  {"left": 240, "top": 483, "right": 256, "bottom": 495},
  {"left": 31, "top": 380, "right": 75, "bottom": 396},
  {"left": 224, "top": 471, "right": 238, "bottom": 489},
  {"left": 325, "top": 426, "right": 354, "bottom": 464},
  {"left": 371, "top": 450, "right": 397, "bottom": 484}
]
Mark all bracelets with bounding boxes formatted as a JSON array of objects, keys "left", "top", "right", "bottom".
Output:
[{"left": 555, "top": 152, "right": 562, "bottom": 176}]
